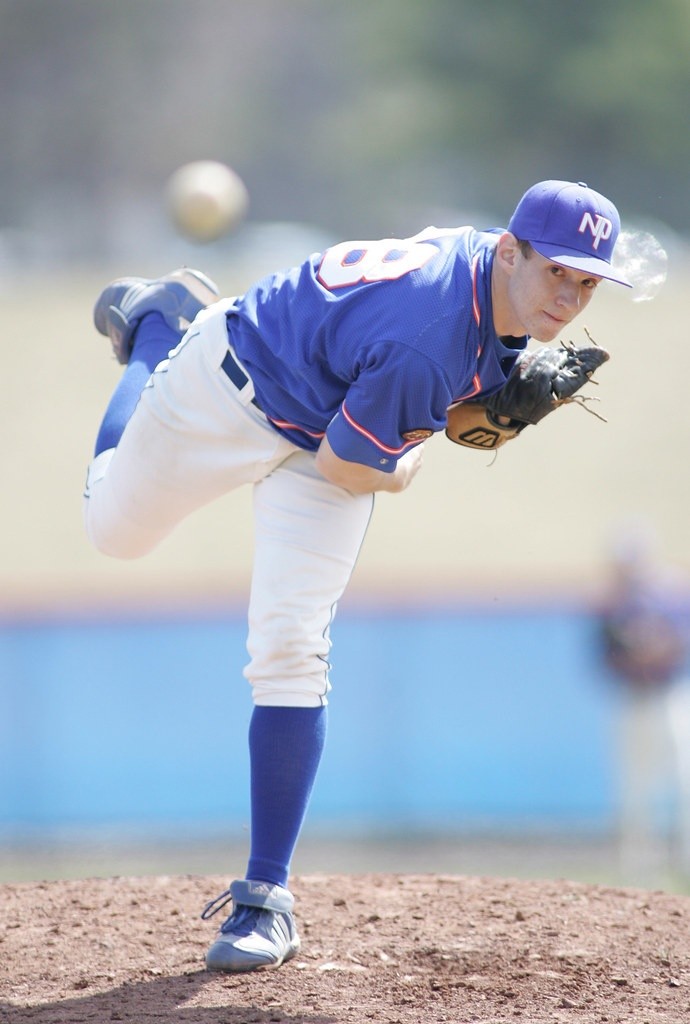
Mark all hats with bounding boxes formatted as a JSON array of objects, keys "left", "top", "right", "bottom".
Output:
[{"left": 508, "top": 180, "right": 634, "bottom": 289}]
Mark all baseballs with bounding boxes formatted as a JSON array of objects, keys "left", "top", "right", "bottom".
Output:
[{"left": 166, "top": 160, "right": 249, "bottom": 246}]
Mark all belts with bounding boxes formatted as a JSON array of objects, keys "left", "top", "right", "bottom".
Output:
[{"left": 221, "top": 350, "right": 265, "bottom": 413}]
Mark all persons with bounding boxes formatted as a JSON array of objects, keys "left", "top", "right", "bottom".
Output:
[
  {"left": 85, "top": 179, "right": 634, "bottom": 971},
  {"left": 601, "top": 552, "right": 690, "bottom": 866}
]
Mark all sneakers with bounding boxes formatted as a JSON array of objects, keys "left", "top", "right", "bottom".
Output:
[
  {"left": 94, "top": 268, "right": 220, "bottom": 365},
  {"left": 201, "top": 879, "right": 301, "bottom": 974}
]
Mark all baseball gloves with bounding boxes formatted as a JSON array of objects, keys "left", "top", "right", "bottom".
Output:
[{"left": 444, "top": 345, "right": 612, "bottom": 449}]
